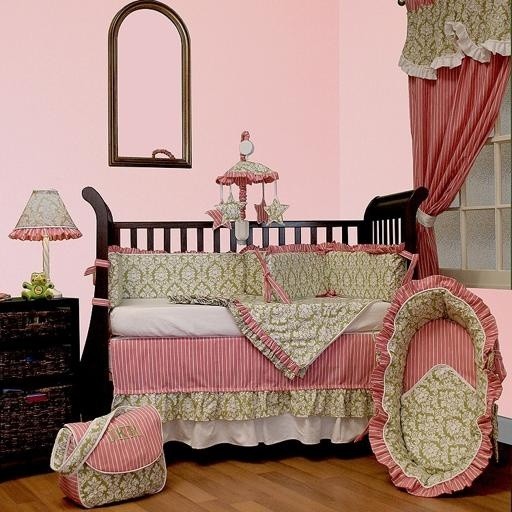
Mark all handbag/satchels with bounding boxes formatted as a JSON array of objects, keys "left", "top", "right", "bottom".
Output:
[{"left": 50, "top": 403, "right": 167, "bottom": 509}]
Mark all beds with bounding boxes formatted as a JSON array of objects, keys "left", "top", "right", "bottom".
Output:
[{"left": 69, "top": 186, "right": 431, "bottom": 463}]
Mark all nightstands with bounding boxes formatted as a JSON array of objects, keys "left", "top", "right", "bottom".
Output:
[{"left": 1, "top": 296, "right": 84, "bottom": 470}]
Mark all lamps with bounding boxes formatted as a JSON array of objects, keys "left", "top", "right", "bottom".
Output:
[{"left": 8, "top": 187, "right": 81, "bottom": 291}]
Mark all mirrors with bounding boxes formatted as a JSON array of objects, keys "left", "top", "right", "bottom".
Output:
[{"left": 106, "top": 1, "right": 196, "bottom": 173}]
